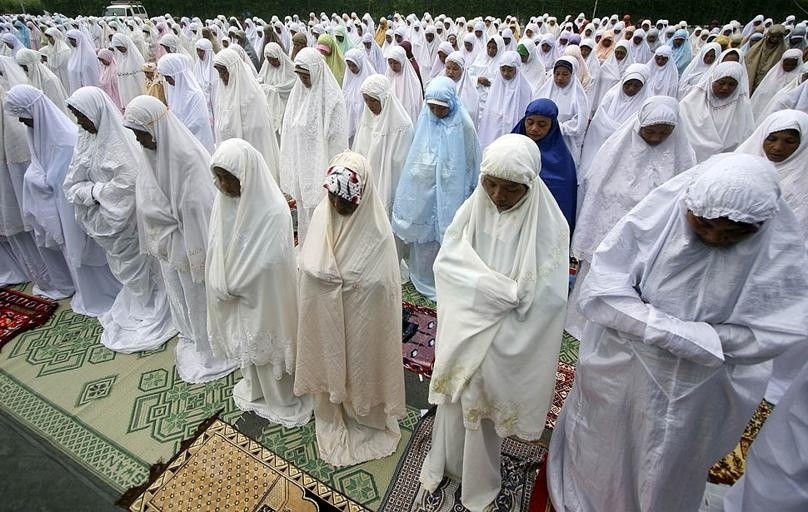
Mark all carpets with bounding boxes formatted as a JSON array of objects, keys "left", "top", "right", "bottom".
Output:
[{"left": 2, "top": 276, "right": 778, "bottom": 512}]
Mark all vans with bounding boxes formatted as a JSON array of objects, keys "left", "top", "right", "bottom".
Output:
[{"left": 102, "top": 0, "right": 151, "bottom": 19}]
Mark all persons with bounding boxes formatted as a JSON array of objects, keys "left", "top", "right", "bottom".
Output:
[
  {"left": 351, "top": 73, "right": 415, "bottom": 283},
  {"left": 291, "top": 150, "right": 407, "bottom": 468},
  {"left": 391, "top": 74, "right": 482, "bottom": 302},
  {"left": 722, "top": 361, "right": 807, "bottom": 510},
  {"left": 420, "top": 134, "right": 570, "bottom": 511},
  {"left": 213, "top": 48, "right": 279, "bottom": 191},
  {"left": 2, "top": 10, "right": 807, "bottom": 203},
  {"left": 0, "top": 57, "right": 78, "bottom": 299},
  {"left": 4, "top": 84, "right": 124, "bottom": 318},
  {"left": 511, "top": 98, "right": 578, "bottom": 244},
  {"left": 563, "top": 96, "right": 698, "bottom": 342},
  {"left": 2, "top": 153, "right": 32, "bottom": 286},
  {"left": 63, "top": 85, "right": 179, "bottom": 355},
  {"left": 547, "top": 151, "right": 808, "bottom": 511},
  {"left": 124, "top": 94, "right": 240, "bottom": 385},
  {"left": 157, "top": 53, "right": 216, "bottom": 160},
  {"left": 205, "top": 138, "right": 313, "bottom": 427},
  {"left": 279, "top": 47, "right": 348, "bottom": 270},
  {"left": 736, "top": 108, "right": 808, "bottom": 407}
]
